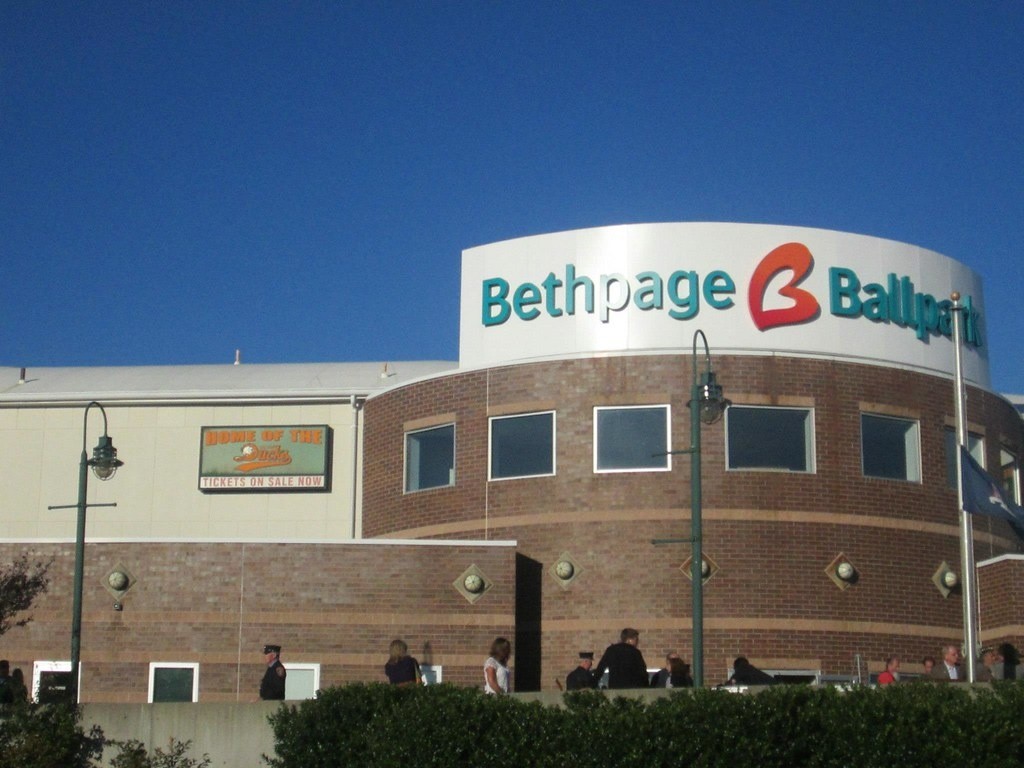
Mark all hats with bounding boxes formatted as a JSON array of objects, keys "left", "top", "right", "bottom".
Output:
[
  {"left": 262, "top": 644, "right": 281, "bottom": 655},
  {"left": 579, "top": 652, "right": 594, "bottom": 660}
]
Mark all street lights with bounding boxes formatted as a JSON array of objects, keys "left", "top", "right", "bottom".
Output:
[
  {"left": 685, "top": 328, "right": 733, "bottom": 689},
  {"left": 47, "top": 401, "right": 126, "bottom": 704}
]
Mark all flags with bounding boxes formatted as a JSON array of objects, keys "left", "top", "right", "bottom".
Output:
[{"left": 960, "top": 444, "right": 1024, "bottom": 529}]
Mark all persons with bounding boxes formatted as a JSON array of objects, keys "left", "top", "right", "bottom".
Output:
[
  {"left": 0, "top": 660, "right": 28, "bottom": 705},
  {"left": 567, "top": 652, "right": 599, "bottom": 689},
  {"left": 647, "top": 652, "right": 694, "bottom": 690},
  {"left": 477, "top": 637, "right": 511, "bottom": 695},
  {"left": 973, "top": 648, "right": 997, "bottom": 682},
  {"left": 878, "top": 656, "right": 901, "bottom": 685},
  {"left": 383, "top": 640, "right": 421, "bottom": 688},
  {"left": 726, "top": 656, "right": 751, "bottom": 685},
  {"left": 593, "top": 628, "right": 651, "bottom": 689},
  {"left": 992, "top": 641, "right": 1024, "bottom": 682},
  {"left": 929, "top": 644, "right": 968, "bottom": 682},
  {"left": 256, "top": 643, "right": 287, "bottom": 700},
  {"left": 919, "top": 655, "right": 935, "bottom": 681}
]
